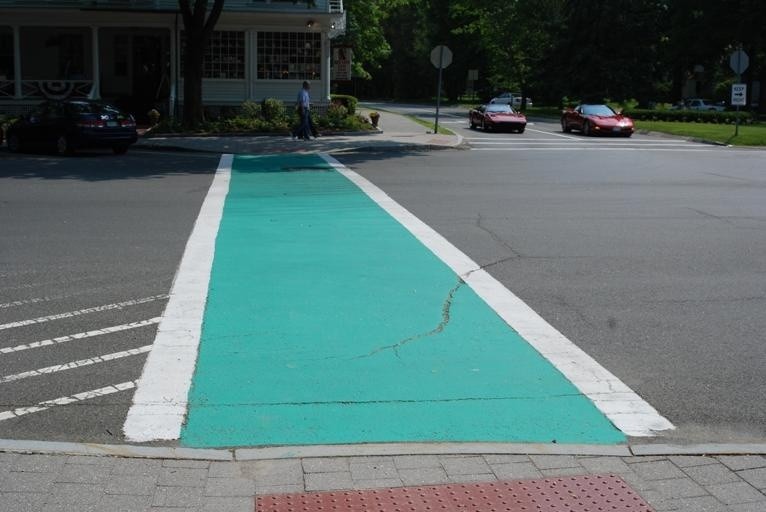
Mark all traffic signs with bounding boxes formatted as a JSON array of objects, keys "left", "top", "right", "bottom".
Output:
[{"left": 732, "top": 84, "right": 747, "bottom": 106}]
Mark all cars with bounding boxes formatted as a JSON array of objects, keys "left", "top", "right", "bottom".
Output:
[
  {"left": 3, "top": 97, "right": 139, "bottom": 158},
  {"left": 669, "top": 98, "right": 728, "bottom": 113},
  {"left": 488, "top": 92, "right": 534, "bottom": 109}
]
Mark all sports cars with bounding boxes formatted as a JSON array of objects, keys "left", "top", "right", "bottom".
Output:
[
  {"left": 468, "top": 102, "right": 528, "bottom": 134},
  {"left": 560, "top": 102, "right": 635, "bottom": 137}
]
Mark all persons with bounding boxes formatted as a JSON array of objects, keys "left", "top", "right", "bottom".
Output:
[
  {"left": 143, "top": 38, "right": 178, "bottom": 120},
  {"left": 292, "top": 105, "right": 322, "bottom": 139},
  {"left": 182, "top": 39, "right": 201, "bottom": 114},
  {"left": 292, "top": 80, "right": 315, "bottom": 141}
]
